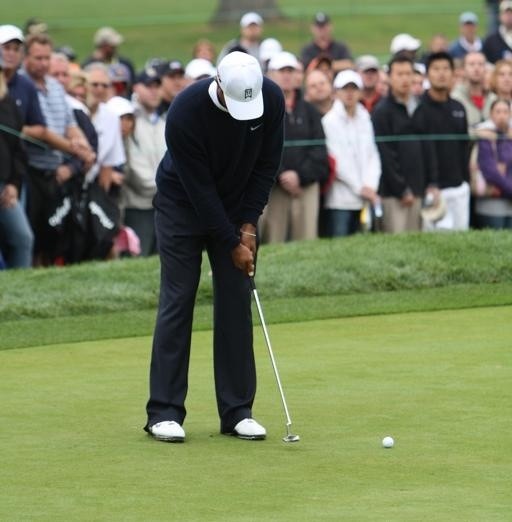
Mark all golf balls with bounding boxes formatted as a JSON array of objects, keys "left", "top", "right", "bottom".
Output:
[{"left": 380, "top": 435, "right": 396, "bottom": 450}]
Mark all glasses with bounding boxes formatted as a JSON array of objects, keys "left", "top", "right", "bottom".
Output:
[{"left": 87, "top": 82, "right": 108, "bottom": 89}]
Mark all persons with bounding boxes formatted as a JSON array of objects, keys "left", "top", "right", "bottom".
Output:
[
  {"left": 0, "top": 18, "right": 218, "bottom": 270},
  {"left": 143, "top": 49, "right": 286, "bottom": 442},
  {"left": 218, "top": 0, "right": 512, "bottom": 243}
]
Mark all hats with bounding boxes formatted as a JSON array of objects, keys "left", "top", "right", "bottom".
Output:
[
  {"left": 108, "top": 62, "right": 130, "bottom": 87},
  {"left": 93, "top": 26, "right": 125, "bottom": 49},
  {"left": 310, "top": 10, "right": 330, "bottom": 27},
  {"left": 240, "top": 11, "right": 265, "bottom": 27},
  {"left": 130, "top": 50, "right": 382, "bottom": 92},
  {"left": 459, "top": 10, "right": 480, "bottom": 26},
  {"left": 390, "top": 33, "right": 423, "bottom": 56},
  {"left": 0, "top": 23, "right": 25, "bottom": 46},
  {"left": 498, "top": 0, "right": 512, "bottom": 12},
  {"left": 215, "top": 51, "right": 266, "bottom": 121}
]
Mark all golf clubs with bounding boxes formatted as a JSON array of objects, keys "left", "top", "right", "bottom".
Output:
[{"left": 248, "top": 272, "right": 300, "bottom": 442}]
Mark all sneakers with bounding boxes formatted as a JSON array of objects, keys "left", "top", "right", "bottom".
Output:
[
  {"left": 225, "top": 417, "right": 267, "bottom": 440},
  {"left": 147, "top": 418, "right": 187, "bottom": 443}
]
